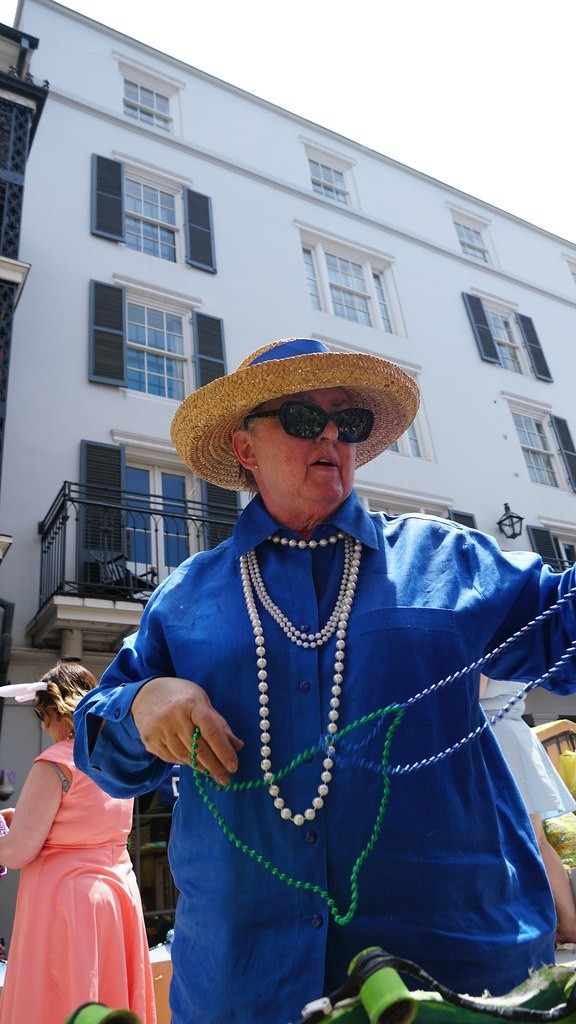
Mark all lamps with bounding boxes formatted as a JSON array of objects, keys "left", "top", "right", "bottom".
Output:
[{"left": 495, "top": 502, "right": 523, "bottom": 538}]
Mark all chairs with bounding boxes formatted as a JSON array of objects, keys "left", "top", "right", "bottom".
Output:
[{"left": 93, "top": 552, "right": 159, "bottom": 598}]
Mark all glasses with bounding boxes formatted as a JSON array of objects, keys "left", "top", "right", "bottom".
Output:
[
  {"left": 244, "top": 400, "right": 374, "bottom": 443},
  {"left": 35, "top": 708, "right": 44, "bottom": 721}
]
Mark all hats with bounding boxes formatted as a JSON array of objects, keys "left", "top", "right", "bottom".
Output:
[{"left": 169, "top": 337, "right": 421, "bottom": 492}]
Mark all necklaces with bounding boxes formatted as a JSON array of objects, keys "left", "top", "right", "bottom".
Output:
[
  {"left": 318, "top": 588, "right": 576, "bottom": 774},
  {"left": 239, "top": 532, "right": 362, "bottom": 826},
  {"left": 190, "top": 705, "right": 404, "bottom": 926}
]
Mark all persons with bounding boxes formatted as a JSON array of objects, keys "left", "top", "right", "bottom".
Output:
[
  {"left": 0, "top": 662, "right": 158, "bottom": 1024},
  {"left": 478, "top": 673, "right": 576, "bottom": 943},
  {"left": 72, "top": 339, "right": 576, "bottom": 1024}
]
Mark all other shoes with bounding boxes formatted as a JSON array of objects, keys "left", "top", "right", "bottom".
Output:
[{"left": 555, "top": 929, "right": 576, "bottom": 950}]
